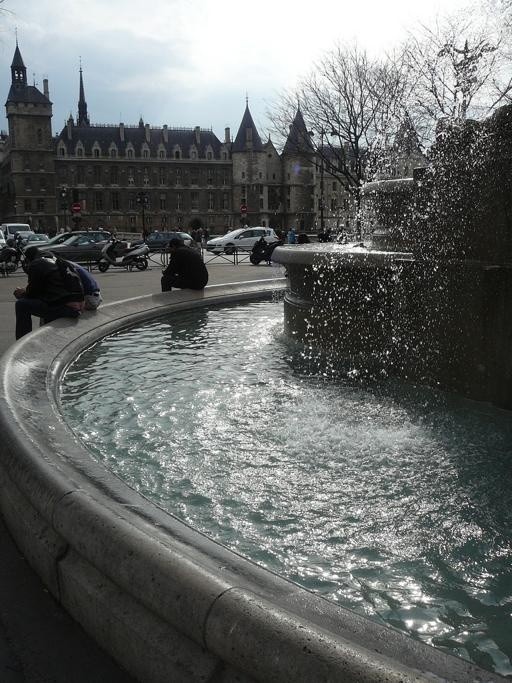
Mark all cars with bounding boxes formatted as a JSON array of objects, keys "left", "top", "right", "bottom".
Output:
[
  {"left": 131, "top": 231, "right": 195, "bottom": 253},
  {"left": 0, "top": 223, "right": 127, "bottom": 265}
]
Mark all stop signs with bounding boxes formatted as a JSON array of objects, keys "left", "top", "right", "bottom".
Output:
[
  {"left": 241, "top": 205, "right": 248, "bottom": 213},
  {"left": 71, "top": 203, "right": 82, "bottom": 213}
]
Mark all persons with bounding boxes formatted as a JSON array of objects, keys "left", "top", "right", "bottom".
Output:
[
  {"left": 272, "top": 223, "right": 310, "bottom": 243},
  {"left": 161, "top": 237, "right": 208, "bottom": 291},
  {"left": 189, "top": 225, "right": 210, "bottom": 242},
  {"left": 13, "top": 246, "right": 85, "bottom": 340},
  {"left": 38, "top": 249, "right": 103, "bottom": 325}
]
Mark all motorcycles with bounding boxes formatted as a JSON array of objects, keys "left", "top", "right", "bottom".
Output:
[
  {"left": 250, "top": 236, "right": 283, "bottom": 266},
  {"left": 0, "top": 232, "right": 29, "bottom": 278},
  {"left": 98, "top": 233, "right": 150, "bottom": 274}
]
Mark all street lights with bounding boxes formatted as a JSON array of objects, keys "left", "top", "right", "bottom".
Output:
[
  {"left": 307, "top": 124, "right": 341, "bottom": 233},
  {"left": 61, "top": 188, "right": 67, "bottom": 232},
  {"left": 137, "top": 192, "right": 149, "bottom": 240},
  {"left": 13, "top": 201, "right": 18, "bottom": 216}
]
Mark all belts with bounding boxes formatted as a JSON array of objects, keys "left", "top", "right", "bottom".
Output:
[{"left": 88, "top": 293, "right": 102, "bottom": 297}]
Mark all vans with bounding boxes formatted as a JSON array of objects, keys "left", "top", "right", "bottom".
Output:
[{"left": 207, "top": 226, "right": 279, "bottom": 255}]
